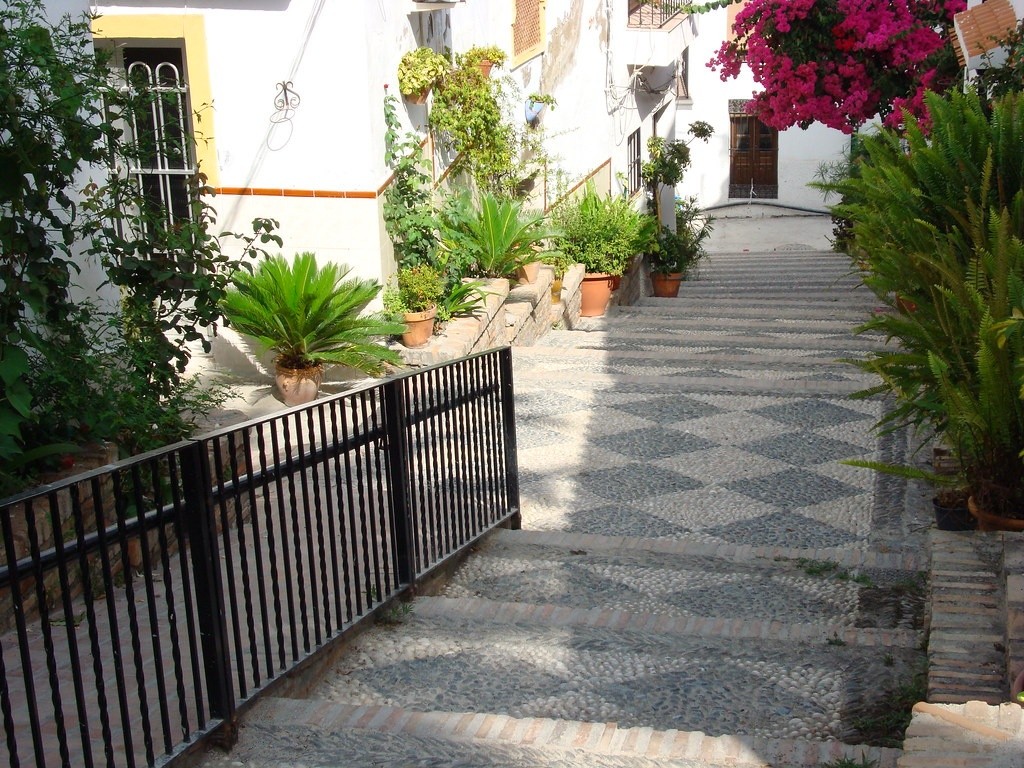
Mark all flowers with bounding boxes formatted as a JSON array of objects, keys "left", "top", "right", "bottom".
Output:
[{"left": 705, "top": 0, "right": 968, "bottom": 142}]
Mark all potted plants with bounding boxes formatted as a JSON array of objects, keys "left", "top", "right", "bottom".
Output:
[
  {"left": 218, "top": 252, "right": 410, "bottom": 407},
  {"left": 644, "top": 224, "right": 695, "bottom": 298},
  {"left": 525, "top": 93, "right": 558, "bottom": 124},
  {"left": 514, "top": 241, "right": 543, "bottom": 285},
  {"left": 545, "top": 252, "right": 574, "bottom": 304},
  {"left": 550, "top": 173, "right": 663, "bottom": 317},
  {"left": 384, "top": 264, "right": 448, "bottom": 348},
  {"left": 809, "top": 87, "right": 1024, "bottom": 532},
  {"left": 398, "top": 45, "right": 453, "bottom": 105},
  {"left": 466, "top": 43, "right": 508, "bottom": 78}
]
